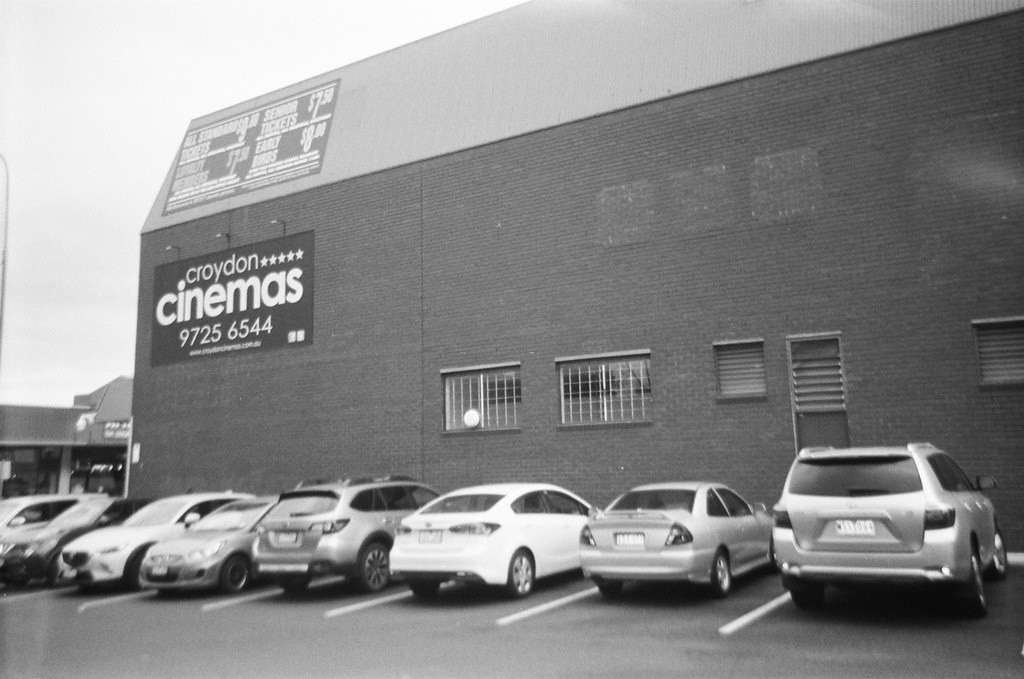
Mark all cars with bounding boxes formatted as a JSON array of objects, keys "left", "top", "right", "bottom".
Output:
[
  {"left": 579, "top": 482, "right": 783, "bottom": 598},
  {"left": 387, "top": 486, "right": 606, "bottom": 600},
  {"left": 0, "top": 490, "right": 148, "bottom": 589},
  {"left": 138, "top": 493, "right": 283, "bottom": 596},
  {"left": 58, "top": 490, "right": 260, "bottom": 593}
]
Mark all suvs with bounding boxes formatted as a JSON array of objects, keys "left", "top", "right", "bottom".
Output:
[
  {"left": 768, "top": 441, "right": 1008, "bottom": 617},
  {"left": 251, "top": 473, "right": 460, "bottom": 594}
]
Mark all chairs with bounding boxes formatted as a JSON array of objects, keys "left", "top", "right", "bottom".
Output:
[
  {"left": 647, "top": 499, "right": 666, "bottom": 509},
  {"left": 482, "top": 498, "right": 497, "bottom": 510},
  {"left": 522, "top": 497, "right": 544, "bottom": 513}
]
